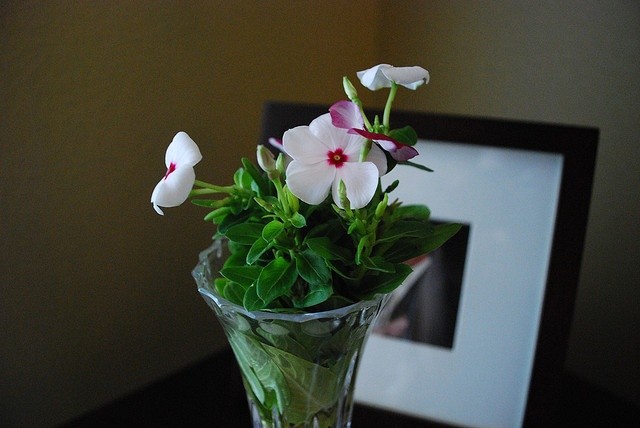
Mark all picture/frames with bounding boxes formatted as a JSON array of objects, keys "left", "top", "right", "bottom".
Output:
[{"left": 259, "top": 101, "right": 601, "bottom": 427}]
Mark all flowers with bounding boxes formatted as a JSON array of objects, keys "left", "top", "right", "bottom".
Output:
[{"left": 151, "top": 64, "right": 463, "bottom": 427}]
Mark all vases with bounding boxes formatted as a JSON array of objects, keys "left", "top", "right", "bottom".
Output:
[{"left": 191, "top": 243, "right": 394, "bottom": 427}]
